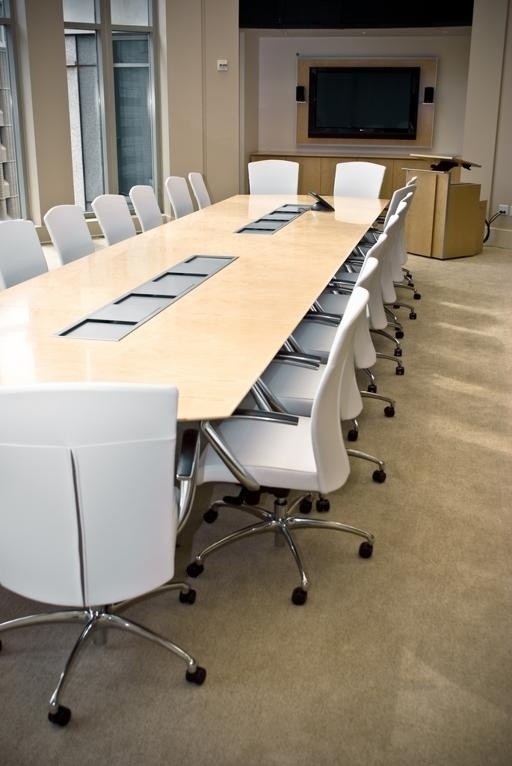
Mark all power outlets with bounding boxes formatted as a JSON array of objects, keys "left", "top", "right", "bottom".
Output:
[{"left": 498, "top": 203, "right": 511, "bottom": 216}]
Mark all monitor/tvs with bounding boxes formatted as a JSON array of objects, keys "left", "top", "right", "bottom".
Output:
[{"left": 307, "top": 66, "right": 420, "bottom": 140}]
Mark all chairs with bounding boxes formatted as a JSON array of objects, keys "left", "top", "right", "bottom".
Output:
[
  {"left": 1, "top": 151, "right": 423, "bottom": 507},
  {"left": 0, "top": 384, "right": 207, "bottom": 728},
  {"left": 182, "top": 288, "right": 374, "bottom": 607}
]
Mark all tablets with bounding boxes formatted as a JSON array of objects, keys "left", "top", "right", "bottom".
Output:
[{"left": 310, "top": 191, "right": 334, "bottom": 210}]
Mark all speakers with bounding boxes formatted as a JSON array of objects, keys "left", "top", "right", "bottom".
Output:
[
  {"left": 296, "top": 86, "right": 304, "bottom": 101},
  {"left": 424, "top": 87, "right": 434, "bottom": 103}
]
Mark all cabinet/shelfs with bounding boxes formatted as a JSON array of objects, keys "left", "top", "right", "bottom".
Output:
[
  {"left": 404, "top": 166, "right": 490, "bottom": 261},
  {"left": 393, "top": 158, "right": 461, "bottom": 193}
]
